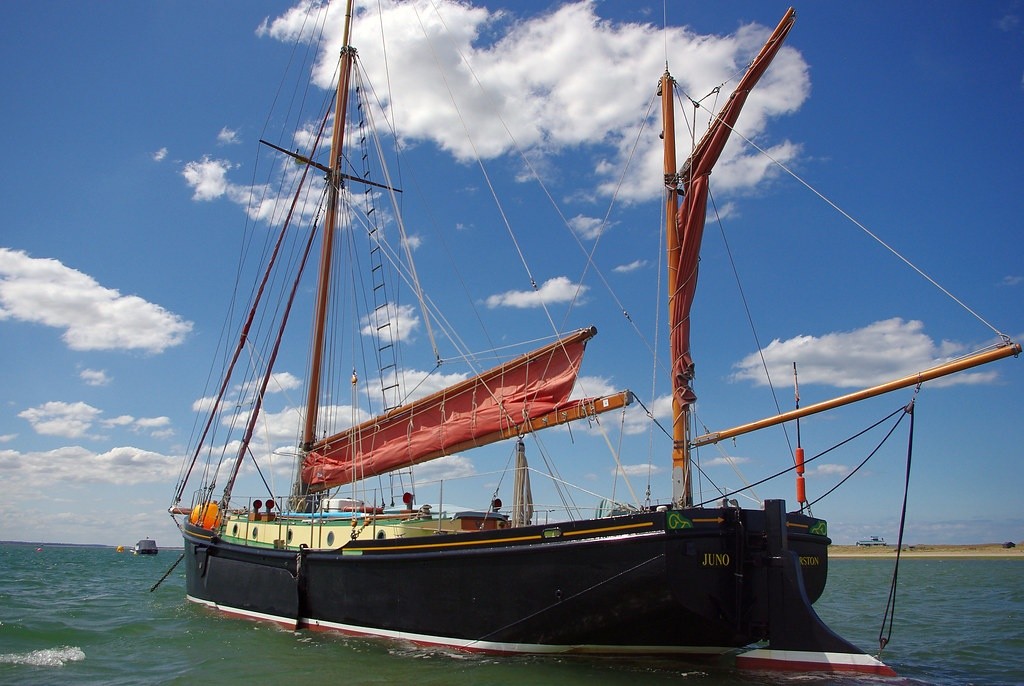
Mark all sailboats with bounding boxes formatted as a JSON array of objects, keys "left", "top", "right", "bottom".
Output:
[{"left": 167, "top": 1, "right": 1020, "bottom": 678}]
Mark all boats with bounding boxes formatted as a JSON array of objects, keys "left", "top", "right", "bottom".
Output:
[
  {"left": 132, "top": 537, "right": 159, "bottom": 557},
  {"left": 857, "top": 535, "right": 887, "bottom": 547}
]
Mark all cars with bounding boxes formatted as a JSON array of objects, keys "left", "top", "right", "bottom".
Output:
[{"left": 1002, "top": 541, "right": 1016, "bottom": 549}]
